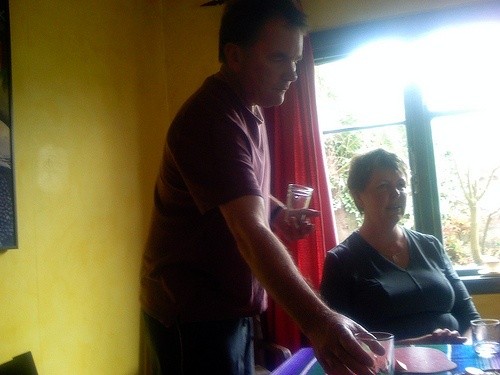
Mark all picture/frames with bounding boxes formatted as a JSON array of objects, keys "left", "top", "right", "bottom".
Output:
[{"left": 0, "top": 1, "right": 19, "bottom": 250}]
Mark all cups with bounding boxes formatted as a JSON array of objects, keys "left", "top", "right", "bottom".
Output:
[
  {"left": 285, "top": 184, "right": 313, "bottom": 229},
  {"left": 470, "top": 318, "right": 500, "bottom": 358},
  {"left": 353, "top": 331, "right": 395, "bottom": 375}
]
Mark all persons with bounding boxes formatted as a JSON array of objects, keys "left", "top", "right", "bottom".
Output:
[
  {"left": 320, "top": 147, "right": 488, "bottom": 344},
  {"left": 137, "top": 0, "right": 387, "bottom": 375}
]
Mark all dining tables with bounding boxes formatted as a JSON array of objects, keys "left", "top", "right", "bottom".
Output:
[{"left": 271, "top": 343, "right": 500, "bottom": 375}]
